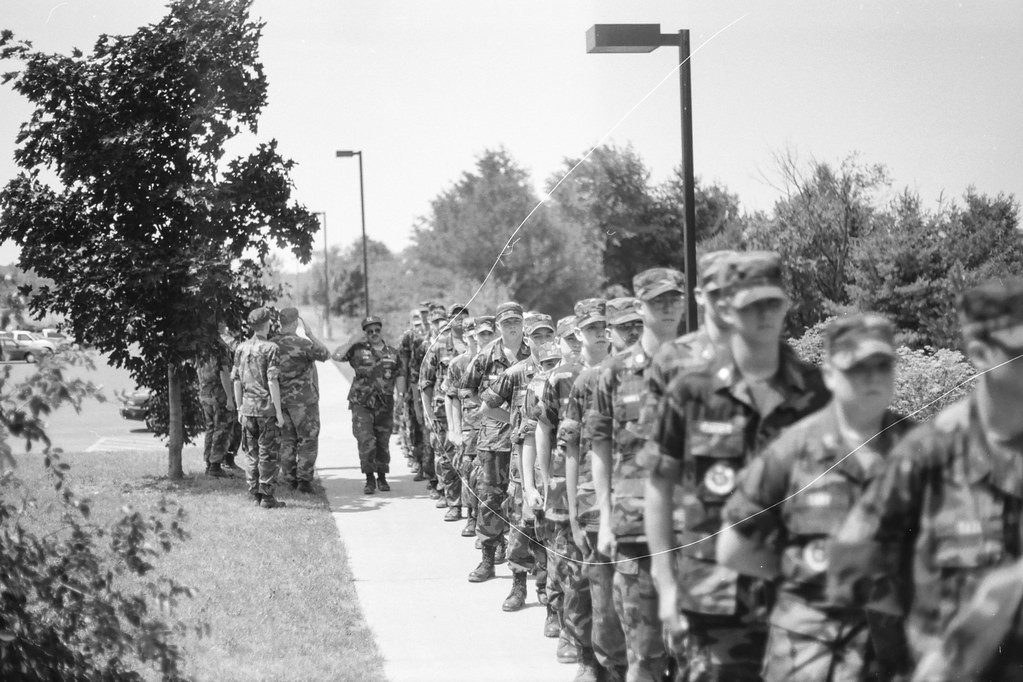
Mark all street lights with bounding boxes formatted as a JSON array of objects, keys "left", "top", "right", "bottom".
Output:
[
  {"left": 336, "top": 151, "right": 371, "bottom": 317},
  {"left": 582, "top": 20, "right": 701, "bottom": 337},
  {"left": 311, "top": 210, "right": 331, "bottom": 340}
]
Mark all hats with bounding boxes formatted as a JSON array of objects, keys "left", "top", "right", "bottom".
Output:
[
  {"left": 496, "top": 302, "right": 523, "bottom": 321},
  {"left": 574, "top": 298, "right": 606, "bottom": 328},
  {"left": 247, "top": 308, "right": 269, "bottom": 324},
  {"left": 632, "top": 268, "right": 685, "bottom": 300},
  {"left": 698, "top": 250, "right": 739, "bottom": 292},
  {"left": 362, "top": 316, "right": 382, "bottom": 330},
  {"left": 827, "top": 313, "right": 898, "bottom": 372},
  {"left": 730, "top": 251, "right": 783, "bottom": 310},
  {"left": 557, "top": 316, "right": 578, "bottom": 338},
  {"left": 409, "top": 301, "right": 495, "bottom": 334},
  {"left": 605, "top": 297, "right": 644, "bottom": 326},
  {"left": 280, "top": 307, "right": 298, "bottom": 323},
  {"left": 539, "top": 342, "right": 562, "bottom": 362},
  {"left": 959, "top": 278, "right": 1023, "bottom": 350},
  {"left": 524, "top": 315, "right": 554, "bottom": 335}
]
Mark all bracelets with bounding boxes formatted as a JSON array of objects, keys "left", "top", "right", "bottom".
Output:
[{"left": 397, "top": 392, "right": 405, "bottom": 397}]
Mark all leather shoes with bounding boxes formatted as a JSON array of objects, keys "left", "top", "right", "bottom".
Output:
[
  {"left": 364, "top": 480, "right": 376, "bottom": 494},
  {"left": 393, "top": 422, "right": 608, "bottom": 682},
  {"left": 206, "top": 455, "right": 315, "bottom": 508},
  {"left": 377, "top": 478, "right": 390, "bottom": 491}
]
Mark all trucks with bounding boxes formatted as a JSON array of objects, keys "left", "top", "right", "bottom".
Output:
[{"left": 33, "top": 329, "right": 73, "bottom": 349}]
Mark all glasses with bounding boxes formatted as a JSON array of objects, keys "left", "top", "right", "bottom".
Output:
[{"left": 367, "top": 328, "right": 380, "bottom": 334}]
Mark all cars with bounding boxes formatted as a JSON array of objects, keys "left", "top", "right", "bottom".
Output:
[{"left": 0, "top": 337, "right": 49, "bottom": 364}]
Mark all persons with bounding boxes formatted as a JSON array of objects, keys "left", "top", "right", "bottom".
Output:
[
  {"left": 643, "top": 250, "right": 833, "bottom": 682},
  {"left": 230, "top": 307, "right": 286, "bottom": 509},
  {"left": 395, "top": 248, "right": 735, "bottom": 682},
  {"left": 193, "top": 307, "right": 331, "bottom": 509},
  {"left": 331, "top": 314, "right": 407, "bottom": 495},
  {"left": 715, "top": 313, "right": 929, "bottom": 682},
  {"left": 828, "top": 278, "right": 1023, "bottom": 682}
]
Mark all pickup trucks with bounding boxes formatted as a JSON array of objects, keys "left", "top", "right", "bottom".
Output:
[{"left": 0, "top": 330, "right": 57, "bottom": 358}]
